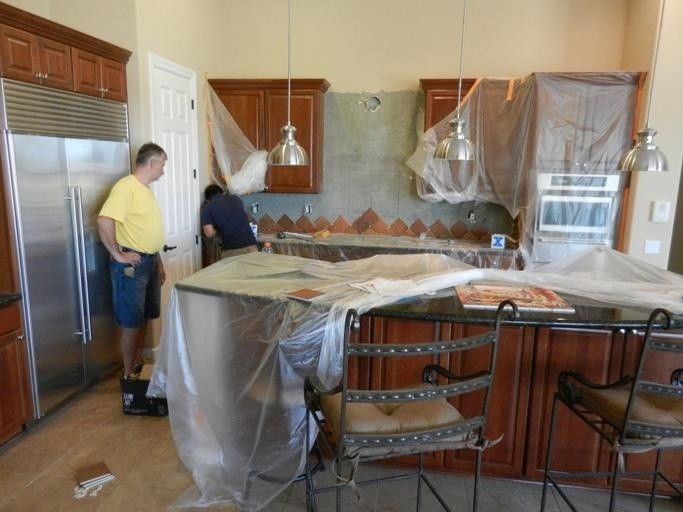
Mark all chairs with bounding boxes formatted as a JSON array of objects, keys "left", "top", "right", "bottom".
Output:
[
  {"left": 550, "top": 301, "right": 679, "bottom": 510},
  {"left": 300, "top": 295, "right": 523, "bottom": 510}
]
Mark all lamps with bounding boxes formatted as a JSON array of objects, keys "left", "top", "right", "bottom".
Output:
[
  {"left": 263, "top": 4, "right": 312, "bottom": 165},
  {"left": 619, "top": 4, "right": 671, "bottom": 175},
  {"left": 431, "top": 1, "right": 484, "bottom": 163}
]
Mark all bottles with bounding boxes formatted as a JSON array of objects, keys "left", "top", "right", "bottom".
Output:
[{"left": 262, "top": 242, "right": 272, "bottom": 252}]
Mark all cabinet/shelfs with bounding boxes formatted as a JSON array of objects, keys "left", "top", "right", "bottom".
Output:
[
  {"left": 372, "top": 313, "right": 534, "bottom": 488},
  {"left": 421, "top": 78, "right": 520, "bottom": 199},
  {"left": 520, "top": 76, "right": 639, "bottom": 171},
  {"left": 1, "top": 22, "right": 73, "bottom": 87},
  {"left": 209, "top": 79, "right": 332, "bottom": 196},
  {"left": 0, "top": 297, "right": 34, "bottom": 437},
  {"left": 224, "top": 302, "right": 370, "bottom": 469},
  {"left": 536, "top": 329, "right": 680, "bottom": 502},
  {"left": 72, "top": 47, "right": 129, "bottom": 107}
]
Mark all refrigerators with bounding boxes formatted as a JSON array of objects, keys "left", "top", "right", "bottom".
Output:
[{"left": 3, "top": 132, "right": 132, "bottom": 421}]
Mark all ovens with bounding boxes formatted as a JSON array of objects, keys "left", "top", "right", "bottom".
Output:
[{"left": 533, "top": 190, "right": 615, "bottom": 242}]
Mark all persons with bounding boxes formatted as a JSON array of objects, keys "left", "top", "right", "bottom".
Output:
[
  {"left": 199, "top": 183, "right": 259, "bottom": 261},
  {"left": 94, "top": 142, "right": 167, "bottom": 388}
]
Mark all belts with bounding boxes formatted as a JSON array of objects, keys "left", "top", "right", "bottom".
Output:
[{"left": 122, "top": 247, "right": 158, "bottom": 257}]
